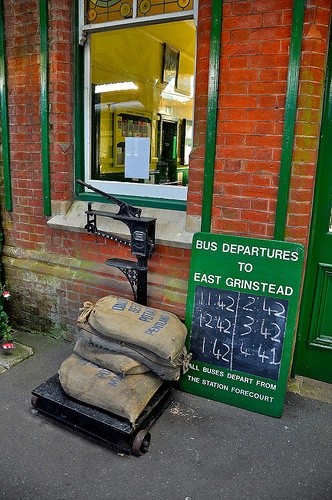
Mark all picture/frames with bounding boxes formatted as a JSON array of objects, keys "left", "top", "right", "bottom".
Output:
[{"left": 162, "top": 42, "right": 181, "bottom": 89}]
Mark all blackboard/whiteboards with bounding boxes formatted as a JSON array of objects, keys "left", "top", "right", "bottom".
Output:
[{"left": 175, "top": 232, "right": 305, "bottom": 420}]
